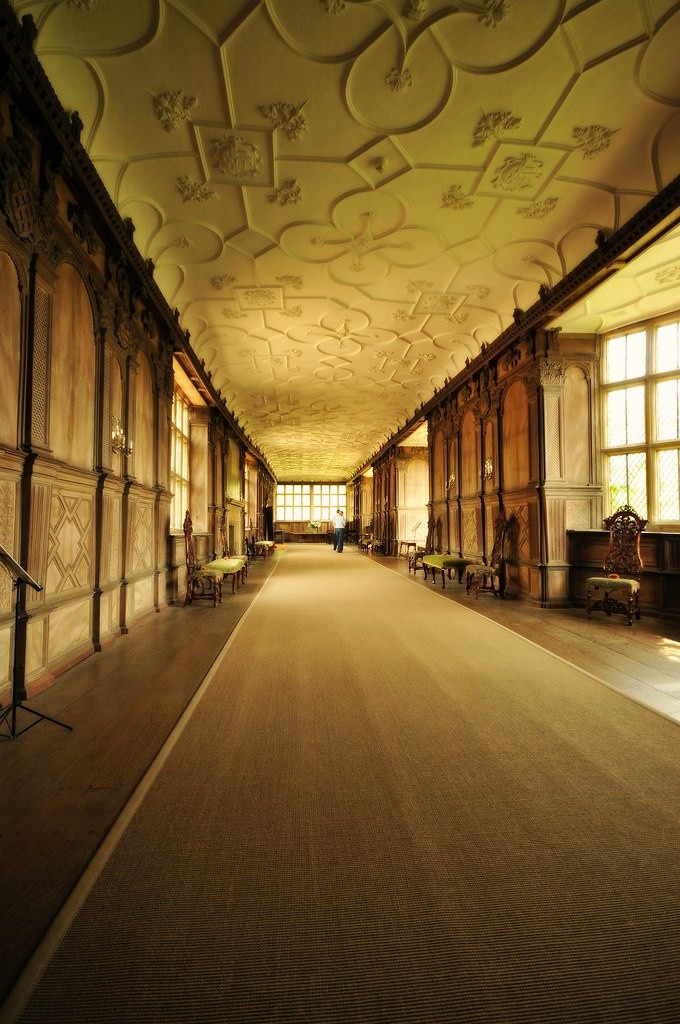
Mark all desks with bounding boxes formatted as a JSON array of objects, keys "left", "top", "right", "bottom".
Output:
[
  {"left": 399, "top": 541, "right": 416, "bottom": 560},
  {"left": 345, "top": 530, "right": 356, "bottom": 544},
  {"left": 274, "top": 530, "right": 283, "bottom": 544}
]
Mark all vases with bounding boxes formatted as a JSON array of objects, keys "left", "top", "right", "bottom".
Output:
[{"left": 313, "top": 527, "right": 317, "bottom": 533}]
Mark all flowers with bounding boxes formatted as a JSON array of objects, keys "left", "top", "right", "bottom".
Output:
[{"left": 307, "top": 521, "right": 321, "bottom": 528}]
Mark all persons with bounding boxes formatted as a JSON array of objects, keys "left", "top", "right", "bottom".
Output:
[
  {"left": 266, "top": 500, "right": 274, "bottom": 538},
  {"left": 333, "top": 510, "right": 346, "bottom": 553}
]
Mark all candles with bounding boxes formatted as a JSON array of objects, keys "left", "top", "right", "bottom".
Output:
[
  {"left": 122, "top": 436, "right": 125, "bottom": 448},
  {"left": 129, "top": 440, "right": 132, "bottom": 450}
]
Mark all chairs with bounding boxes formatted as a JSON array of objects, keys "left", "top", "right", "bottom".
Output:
[
  {"left": 245, "top": 518, "right": 266, "bottom": 561},
  {"left": 408, "top": 517, "right": 436, "bottom": 576},
  {"left": 183, "top": 510, "right": 223, "bottom": 608},
  {"left": 219, "top": 509, "right": 248, "bottom": 584},
  {"left": 358, "top": 519, "right": 374, "bottom": 552},
  {"left": 466, "top": 513, "right": 509, "bottom": 600},
  {"left": 371, "top": 520, "right": 385, "bottom": 556},
  {"left": 585, "top": 505, "right": 649, "bottom": 627}
]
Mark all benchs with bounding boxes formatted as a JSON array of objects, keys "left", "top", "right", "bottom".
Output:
[
  {"left": 422, "top": 555, "right": 471, "bottom": 590},
  {"left": 284, "top": 533, "right": 328, "bottom": 544},
  {"left": 362, "top": 538, "right": 376, "bottom": 555},
  {"left": 201, "top": 558, "right": 246, "bottom": 594},
  {"left": 254, "top": 541, "right": 276, "bottom": 557}
]
